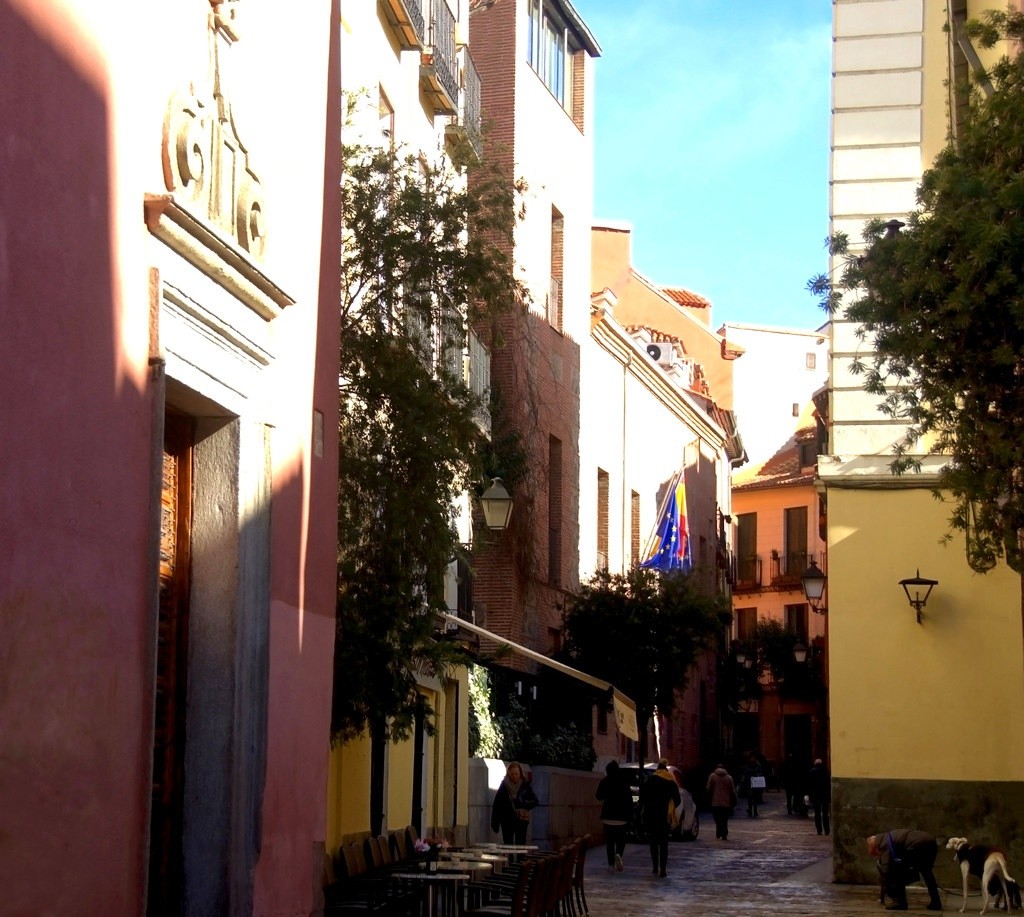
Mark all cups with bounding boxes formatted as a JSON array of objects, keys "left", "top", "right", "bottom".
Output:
[
  {"left": 474, "top": 850, "right": 483, "bottom": 857},
  {"left": 488, "top": 843, "right": 496, "bottom": 851},
  {"left": 451, "top": 858, "right": 461, "bottom": 865}
]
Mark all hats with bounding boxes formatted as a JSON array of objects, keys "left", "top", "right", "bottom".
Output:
[{"left": 866, "top": 833, "right": 875, "bottom": 859}]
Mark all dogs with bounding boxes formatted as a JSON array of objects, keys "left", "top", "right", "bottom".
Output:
[{"left": 945, "top": 837, "right": 1022, "bottom": 917}]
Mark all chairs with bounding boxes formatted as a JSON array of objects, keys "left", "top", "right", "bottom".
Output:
[{"left": 324, "top": 826, "right": 594, "bottom": 917}]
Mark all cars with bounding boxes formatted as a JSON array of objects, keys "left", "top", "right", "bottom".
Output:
[{"left": 622, "top": 760, "right": 700, "bottom": 841}]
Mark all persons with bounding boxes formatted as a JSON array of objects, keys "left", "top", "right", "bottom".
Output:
[
  {"left": 595, "top": 759, "right": 633, "bottom": 872},
  {"left": 705, "top": 764, "right": 735, "bottom": 839},
  {"left": 491, "top": 762, "right": 539, "bottom": 866},
  {"left": 804, "top": 758, "right": 831, "bottom": 836},
  {"left": 736, "top": 756, "right": 767, "bottom": 818},
  {"left": 776, "top": 753, "right": 806, "bottom": 817},
  {"left": 866, "top": 829, "right": 944, "bottom": 912},
  {"left": 638, "top": 758, "right": 681, "bottom": 881}
]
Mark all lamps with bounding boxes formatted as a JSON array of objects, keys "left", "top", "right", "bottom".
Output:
[{"left": 899, "top": 569, "right": 939, "bottom": 622}]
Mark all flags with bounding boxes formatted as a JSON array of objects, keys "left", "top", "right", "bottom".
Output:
[
  {"left": 669, "top": 473, "right": 692, "bottom": 576},
  {"left": 641, "top": 486, "right": 680, "bottom": 574}
]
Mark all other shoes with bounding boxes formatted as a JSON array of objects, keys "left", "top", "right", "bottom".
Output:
[
  {"left": 715, "top": 831, "right": 728, "bottom": 842},
  {"left": 609, "top": 853, "right": 624, "bottom": 875},
  {"left": 927, "top": 896, "right": 942, "bottom": 910},
  {"left": 885, "top": 898, "right": 906, "bottom": 910},
  {"left": 652, "top": 866, "right": 668, "bottom": 879}
]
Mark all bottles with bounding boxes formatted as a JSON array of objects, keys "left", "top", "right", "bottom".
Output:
[{"left": 426, "top": 843, "right": 438, "bottom": 876}]
